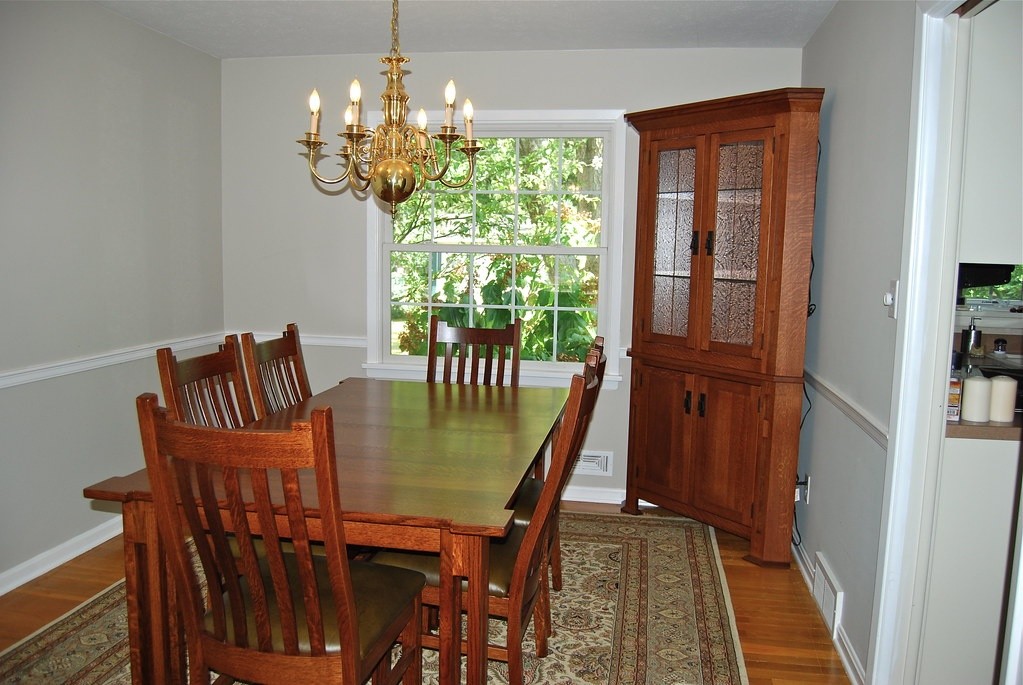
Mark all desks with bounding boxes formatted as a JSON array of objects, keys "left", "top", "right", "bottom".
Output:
[{"left": 83, "top": 377, "right": 569, "bottom": 685}]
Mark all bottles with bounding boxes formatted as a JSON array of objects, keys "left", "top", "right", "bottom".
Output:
[{"left": 946, "top": 365, "right": 963, "bottom": 422}]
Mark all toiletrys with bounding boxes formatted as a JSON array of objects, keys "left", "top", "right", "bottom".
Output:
[{"left": 961, "top": 316, "right": 982, "bottom": 353}]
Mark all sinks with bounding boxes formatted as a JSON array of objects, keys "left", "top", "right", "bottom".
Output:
[{"left": 986, "top": 351, "right": 1022, "bottom": 367}]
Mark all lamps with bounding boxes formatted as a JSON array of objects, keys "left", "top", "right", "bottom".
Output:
[{"left": 296, "top": 0, "right": 486, "bottom": 220}]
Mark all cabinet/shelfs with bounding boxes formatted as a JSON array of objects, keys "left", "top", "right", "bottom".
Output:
[{"left": 623, "top": 88, "right": 826, "bottom": 570}]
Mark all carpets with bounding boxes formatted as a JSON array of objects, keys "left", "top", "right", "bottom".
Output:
[{"left": 0, "top": 509, "right": 750, "bottom": 685}]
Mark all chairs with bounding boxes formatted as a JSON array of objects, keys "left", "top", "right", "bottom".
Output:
[
  {"left": 364, "top": 354, "right": 601, "bottom": 685},
  {"left": 426, "top": 314, "right": 522, "bottom": 387},
  {"left": 156, "top": 334, "right": 362, "bottom": 613},
  {"left": 241, "top": 322, "right": 313, "bottom": 421},
  {"left": 512, "top": 336, "right": 608, "bottom": 638},
  {"left": 135, "top": 392, "right": 426, "bottom": 685}
]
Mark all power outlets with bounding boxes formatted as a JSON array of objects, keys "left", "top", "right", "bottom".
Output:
[
  {"left": 883, "top": 280, "right": 899, "bottom": 319},
  {"left": 803, "top": 472, "right": 811, "bottom": 505}
]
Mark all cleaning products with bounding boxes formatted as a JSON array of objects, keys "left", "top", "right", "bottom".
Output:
[{"left": 945, "top": 364, "right": 962, "bottom": 422}]
